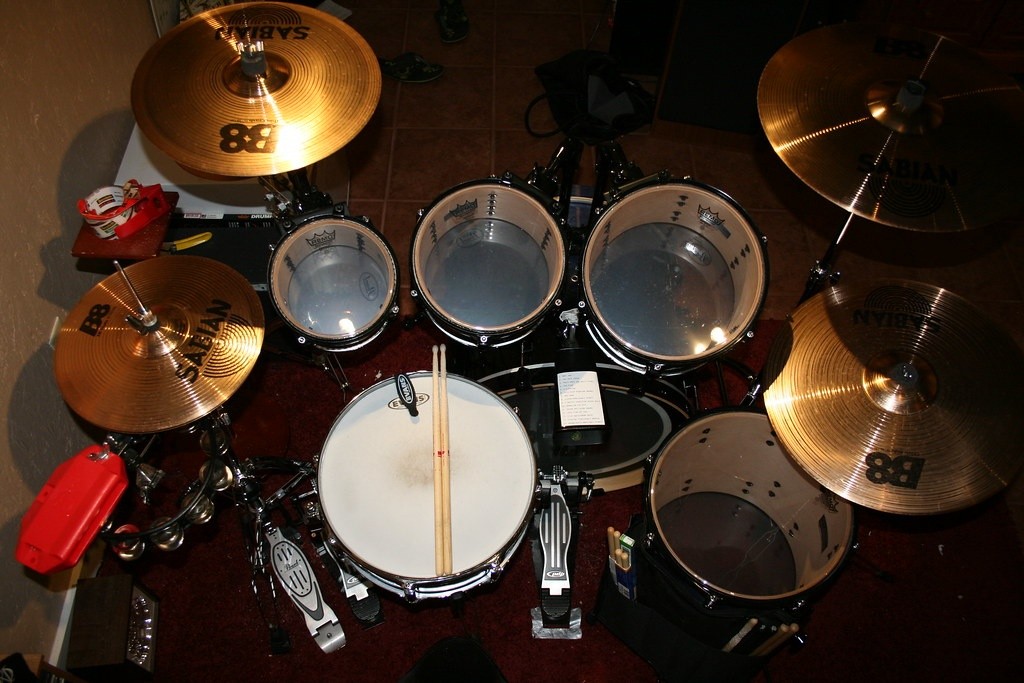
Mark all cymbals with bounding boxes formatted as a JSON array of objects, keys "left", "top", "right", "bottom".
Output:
[
  {"left": 200, "top": 428, "right": 230, "bottom": 457},
  {"left": 761, "top": 277, "right": 1024, "bottom": 516},
  {"left": 111, "top": 524, "right": 145, "bottom": 560},
  {"left": 183, "top": 493, "right": 216, "bottom": 525},
  {"left": 130, "top": 1, "right": 382, "bottom": 178},
  {"left": 150, "top": 516, "right": 185, "bottom": 551},
  {"left": 54, "top": 254, "right": 264, "bottom": 434},
  {"left": 200, "top": 461, "right": 234, "bottom": 492},
  {"left": 756, "top": 12, "right": 1024, "bottom": 233}
]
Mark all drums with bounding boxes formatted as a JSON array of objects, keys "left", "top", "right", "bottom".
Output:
[
  {"left": 438, "top": 192, "right": 694, "bottom": 496},
  {"left": 576, "top": 177, "right": 769, "bottom": 378},
  {"left": 647, "top": 405, "right": 855, "bottom": 611},
  {"left": 409, "top": 178, "right": 566, "bottom": 348},
  {"left": 266, "top": 215, "right": 400, "bottom": 353},
  {"left": 316, "top": 369, "right": 536, "bottom": 601}
]
